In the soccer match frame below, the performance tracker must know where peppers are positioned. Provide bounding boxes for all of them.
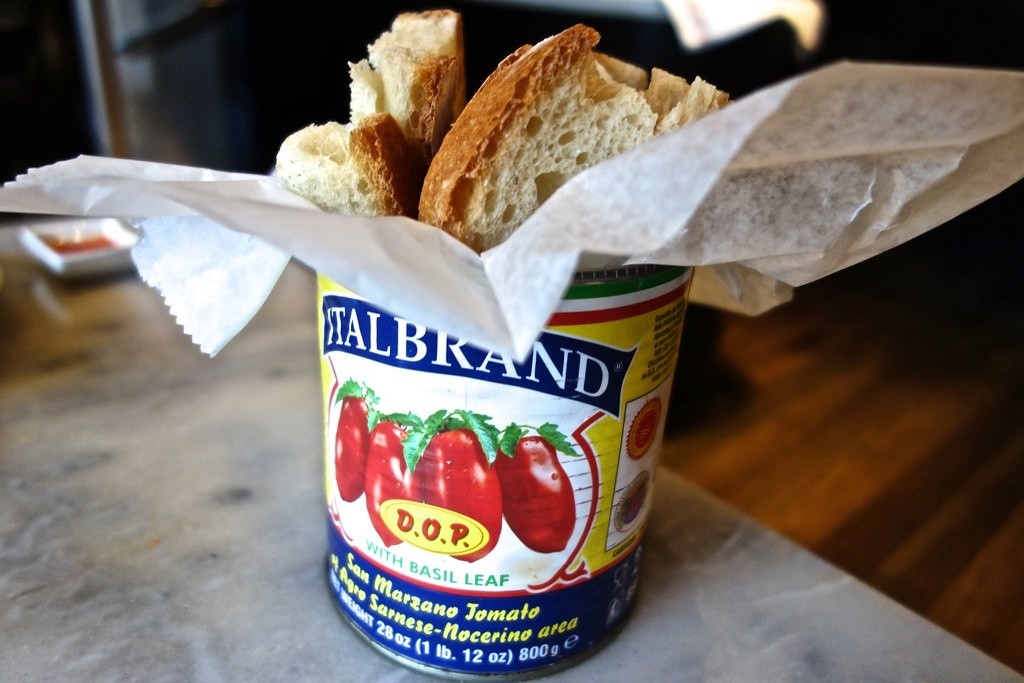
[335,396,576,562]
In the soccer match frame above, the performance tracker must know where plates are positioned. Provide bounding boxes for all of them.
[20,217,141,275]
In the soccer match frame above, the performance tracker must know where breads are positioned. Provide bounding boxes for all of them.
[273,11,733,259]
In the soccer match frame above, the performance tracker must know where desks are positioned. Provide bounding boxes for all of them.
[0,219,1023,683]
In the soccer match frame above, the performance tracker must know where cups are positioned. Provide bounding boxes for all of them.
[316,263,693,683]
